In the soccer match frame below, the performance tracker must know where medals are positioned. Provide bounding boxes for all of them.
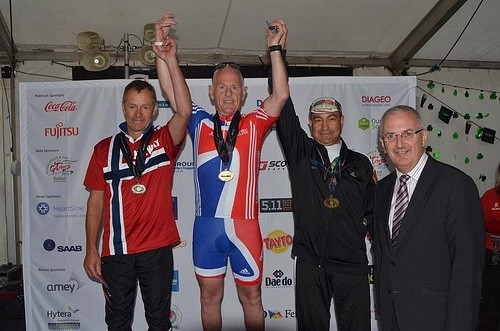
[322,196,339,208]
[218,169,233,181]
[131,183,147,194]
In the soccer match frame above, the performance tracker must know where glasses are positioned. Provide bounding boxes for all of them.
[212,61,243,81]
[381,128,424,142]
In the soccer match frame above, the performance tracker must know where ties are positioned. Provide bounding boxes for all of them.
[392,174,411,250]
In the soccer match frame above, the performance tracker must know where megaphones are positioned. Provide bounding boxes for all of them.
[77,31,111,71]
[139,23,158,65]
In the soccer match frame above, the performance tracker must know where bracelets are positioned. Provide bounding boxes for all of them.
[153,41,164,46]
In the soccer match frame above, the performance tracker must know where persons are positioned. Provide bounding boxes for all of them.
[80,36,199,331]
[479,162,500,279]
[151,11,291,331]
[364,102,485,331]
[266,16,382,331]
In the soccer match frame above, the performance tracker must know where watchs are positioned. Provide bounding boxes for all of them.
[270,44,283,53]
